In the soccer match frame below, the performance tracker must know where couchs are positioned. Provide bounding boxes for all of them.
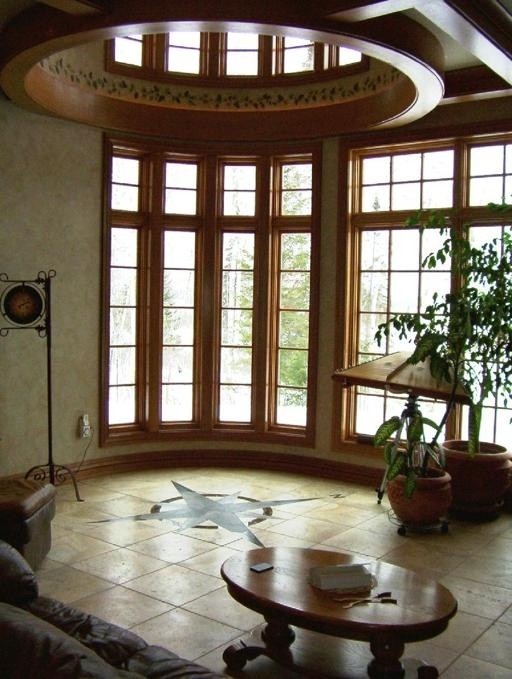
[0,538,224,677]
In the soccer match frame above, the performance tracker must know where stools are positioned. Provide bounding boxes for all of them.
[0,478,57,572]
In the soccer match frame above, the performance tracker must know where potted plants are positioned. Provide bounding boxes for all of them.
[373,408,451,538]
[372,203,512,522]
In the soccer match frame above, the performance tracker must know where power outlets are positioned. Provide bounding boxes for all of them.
[80,426,91,438]
